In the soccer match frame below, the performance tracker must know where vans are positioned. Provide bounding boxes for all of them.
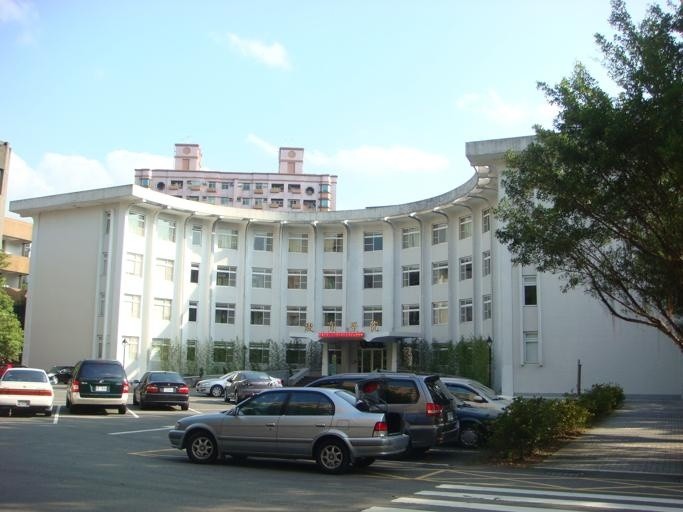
[302,372,463,461]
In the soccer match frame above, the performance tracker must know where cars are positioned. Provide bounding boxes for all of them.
[66,358,130,414]
[45,365,74,385]
[195,370,284,405]
[0,366,55,417]
[132,370,190,410]
[438,376,526,449]
[167,386,410,474]
